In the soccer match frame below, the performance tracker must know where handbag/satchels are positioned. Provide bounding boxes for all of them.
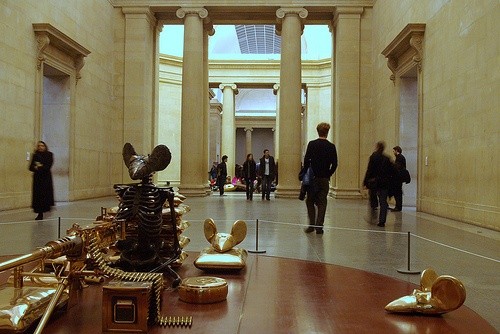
[298,173,309,201]
[404,170,412,184]
[365,174,379,188]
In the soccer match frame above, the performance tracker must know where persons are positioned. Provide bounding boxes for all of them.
[258,150,276,200]
[29,140,54,220]
[242,154,257,200]
[299,123,338,234]
[235,159,278,194]
[364,141,406,227]
[208,155,228,196]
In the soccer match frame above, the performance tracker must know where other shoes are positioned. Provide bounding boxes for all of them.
[316,227,323,234]
[377,223,386,227]
[391,207,401,211]
[305,227,314,232]
[35,212,44,220]
